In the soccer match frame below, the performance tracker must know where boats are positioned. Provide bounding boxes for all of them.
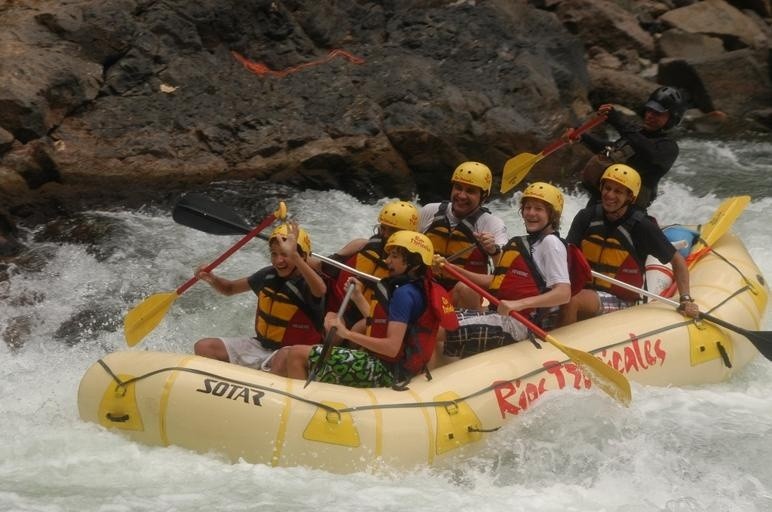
[77,224,771,479]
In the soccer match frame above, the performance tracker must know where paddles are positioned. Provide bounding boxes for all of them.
[304,277,356,389]
[124,201,288,348]
[591,269,772,367]
[432,254,632,411]
[501,109,609,195]
[173,192,383,287]
[650,195,750,303]
[445,239,481,267]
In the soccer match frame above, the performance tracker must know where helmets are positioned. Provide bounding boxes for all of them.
[600,163,642,198]
[269,225,310,261]
[377,202,423,234]
[520,182,564,215]
[451,161,492,197]
[646,86,683,129]
[384,231,433,266]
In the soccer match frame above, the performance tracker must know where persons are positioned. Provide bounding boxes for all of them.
[307,201,420,347]
[558,163,699,329]
[193,218,327,378]
[418,161,508,311]
[561,86,685,215]
[271,230,459,388]
[431,182,571,368]
